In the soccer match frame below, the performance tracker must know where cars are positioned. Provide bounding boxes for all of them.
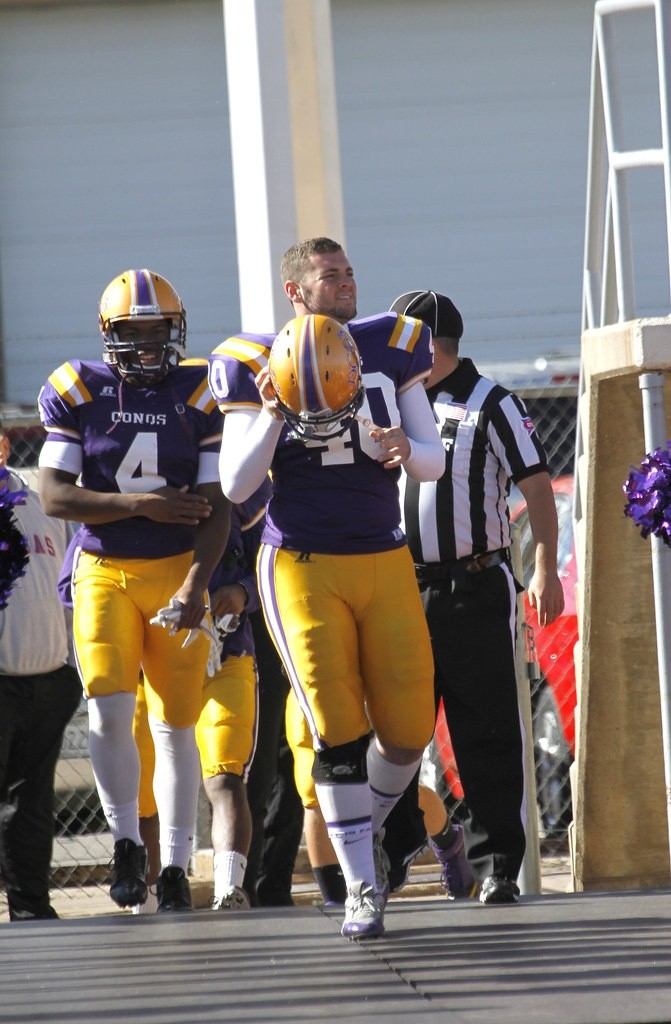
[505,475,577,621]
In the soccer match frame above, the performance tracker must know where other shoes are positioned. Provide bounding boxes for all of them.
[398,808,429,865]
[477,873,520,904]
[391,856,414,891]
[7,900,59,922]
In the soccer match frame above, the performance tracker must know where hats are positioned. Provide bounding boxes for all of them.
[389,289,464,339]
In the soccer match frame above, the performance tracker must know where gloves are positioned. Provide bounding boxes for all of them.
[150,598,224,678]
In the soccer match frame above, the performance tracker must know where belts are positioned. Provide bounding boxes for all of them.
[414,550,507,582]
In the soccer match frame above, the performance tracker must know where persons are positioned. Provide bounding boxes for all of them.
[0,268,565,922]
[206,237,446,942]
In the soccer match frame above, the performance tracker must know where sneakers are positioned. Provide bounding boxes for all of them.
[371,827,391,904]
[341,883,383,940]
[208,885,251,910]
[107,839,148,908]
[321,899,344,912]
[433,825,476,899]
[149,865,194,912]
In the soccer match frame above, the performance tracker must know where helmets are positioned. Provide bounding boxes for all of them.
[97,269,187,387]
[268,314,364,440]
